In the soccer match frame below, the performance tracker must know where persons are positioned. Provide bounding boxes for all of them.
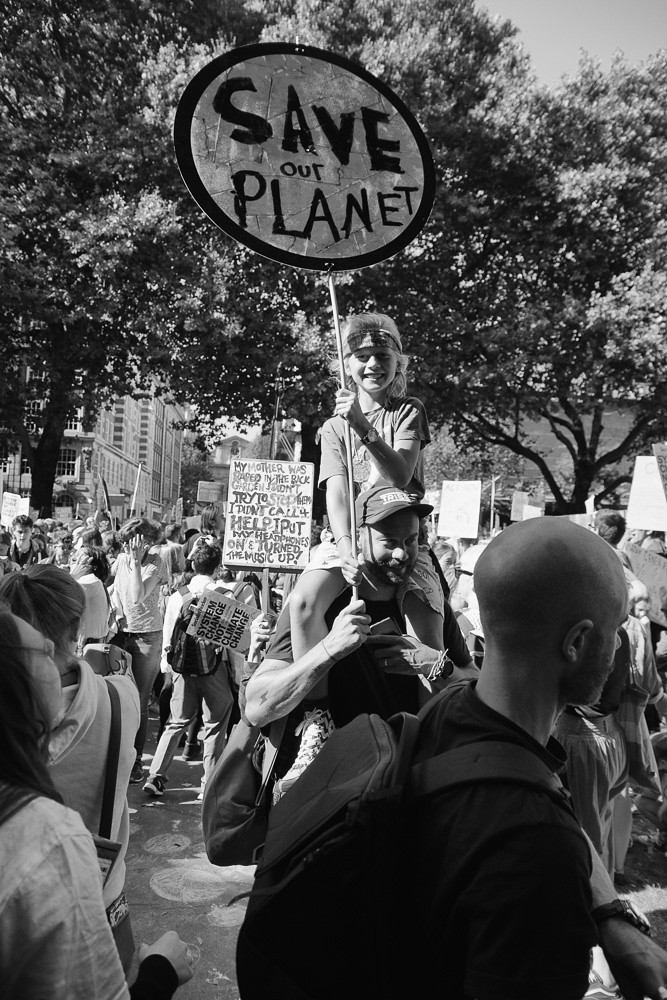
[232,518,629,1000]
[1,606,194,1000]
[2,502,652,993]
[270,310,447,810]
[1,564,146,988]
[237,481,482,814]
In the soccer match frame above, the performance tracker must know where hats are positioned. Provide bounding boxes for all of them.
[355,486,434,528]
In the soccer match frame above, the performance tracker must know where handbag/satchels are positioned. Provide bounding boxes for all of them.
[201,719,270,866]
[614,657,663,795]
[91,833,124,891]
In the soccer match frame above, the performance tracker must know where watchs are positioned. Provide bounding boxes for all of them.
[593,899,652,938]
[432,650,456,683]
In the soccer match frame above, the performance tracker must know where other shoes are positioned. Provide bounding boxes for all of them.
[182,740,200,761]
[130,761,144,782]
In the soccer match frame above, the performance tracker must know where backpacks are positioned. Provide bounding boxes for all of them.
[166,588,234,678]
[235,681,574,1000]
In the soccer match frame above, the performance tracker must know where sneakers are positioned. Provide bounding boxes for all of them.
[273,707,339,794]
[142,778,165,795]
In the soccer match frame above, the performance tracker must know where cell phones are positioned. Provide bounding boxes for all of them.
[369,617,403,647]
